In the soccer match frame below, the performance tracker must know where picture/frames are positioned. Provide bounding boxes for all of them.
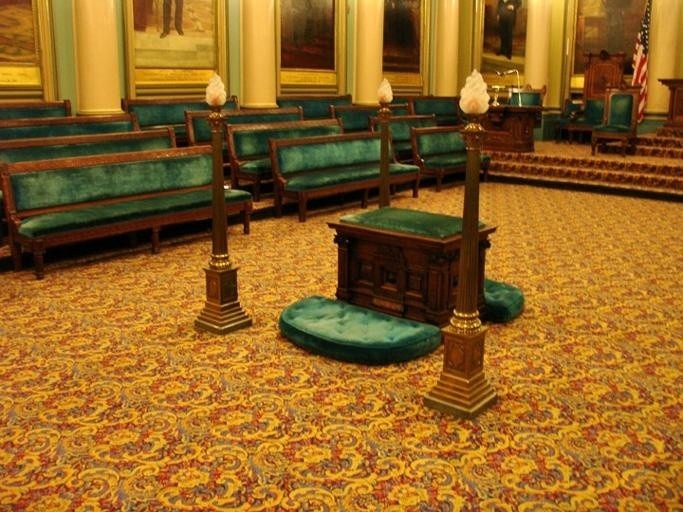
[571,0,645,78]
[1,0,61,106]
[274,0,346,98]
[381,0,430,97]
[477,0,529,85]
[123,0,230,102]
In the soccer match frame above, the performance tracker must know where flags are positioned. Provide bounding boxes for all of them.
[631,1,651,126]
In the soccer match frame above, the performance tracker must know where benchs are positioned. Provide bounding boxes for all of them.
[120,92,489,224]
[0,98,253,281]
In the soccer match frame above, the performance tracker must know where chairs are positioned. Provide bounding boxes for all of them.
[506,84,639,159]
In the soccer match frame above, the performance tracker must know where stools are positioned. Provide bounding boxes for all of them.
[327,205,497,329]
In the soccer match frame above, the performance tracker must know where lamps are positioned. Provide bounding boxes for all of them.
[192,71,253,336]
[420,69,498,418]
[497,68,522,106]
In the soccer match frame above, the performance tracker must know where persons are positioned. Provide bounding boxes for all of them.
[496,0,522,60]
[159,0,185,38]
